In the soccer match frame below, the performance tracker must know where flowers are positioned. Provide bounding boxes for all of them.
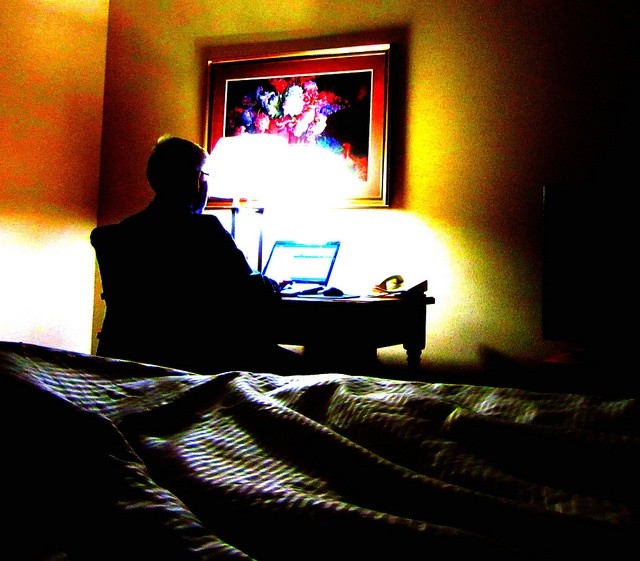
[232,81,355,146]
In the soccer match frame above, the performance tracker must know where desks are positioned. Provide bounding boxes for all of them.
[284,292,437,375]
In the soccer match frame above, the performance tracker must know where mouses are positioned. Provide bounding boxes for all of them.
[322,287,343,296]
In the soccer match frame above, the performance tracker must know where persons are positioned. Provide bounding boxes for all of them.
[117,137,302,376]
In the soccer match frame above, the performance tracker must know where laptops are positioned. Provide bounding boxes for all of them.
[264,239,341,297]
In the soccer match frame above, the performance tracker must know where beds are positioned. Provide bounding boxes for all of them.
[0,341,638,555]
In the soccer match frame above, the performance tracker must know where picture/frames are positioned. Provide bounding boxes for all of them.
[201,43,394,212]
